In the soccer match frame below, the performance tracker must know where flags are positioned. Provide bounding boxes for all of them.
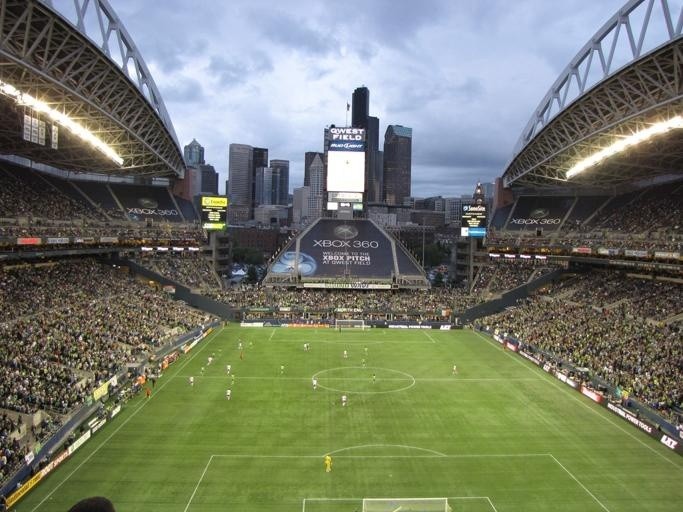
[347,104,350,111]
